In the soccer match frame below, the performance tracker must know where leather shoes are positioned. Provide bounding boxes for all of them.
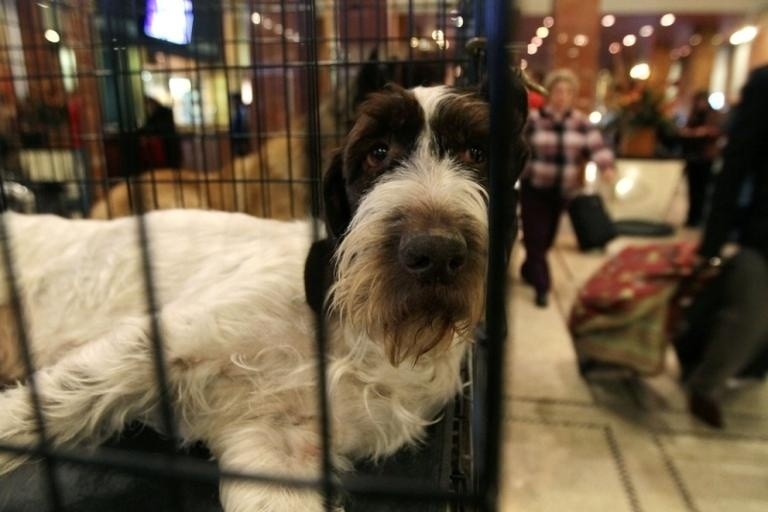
[534,289,546,307]
[688,389,728,433]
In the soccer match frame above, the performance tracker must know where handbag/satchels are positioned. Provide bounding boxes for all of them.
[563,192,618,254]
[566,243,697,379]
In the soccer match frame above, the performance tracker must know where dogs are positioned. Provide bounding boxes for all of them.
[0,74,531,512]
[90,77,354,221]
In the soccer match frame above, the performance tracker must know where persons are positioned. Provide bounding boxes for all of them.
[134,96,188,168]
[228,92,254,158]
[518,66,768,430]
